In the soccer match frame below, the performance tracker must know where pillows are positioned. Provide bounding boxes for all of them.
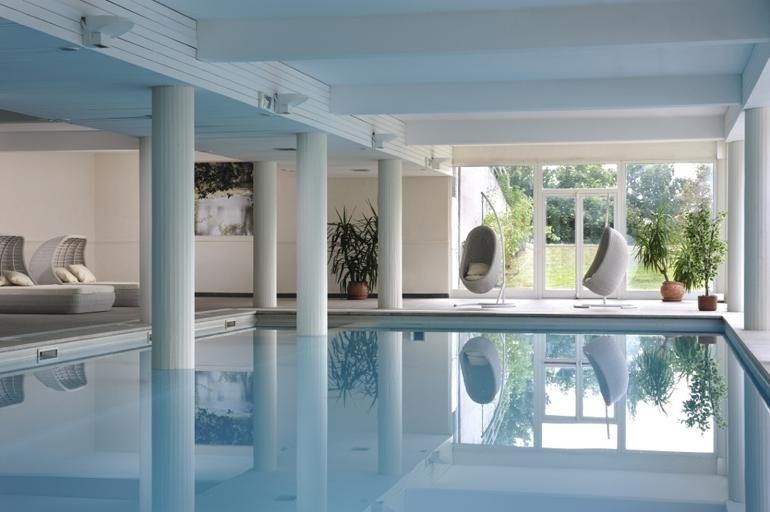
[466,260,488,280]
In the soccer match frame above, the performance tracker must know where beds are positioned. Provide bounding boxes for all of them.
[0,233,140,314]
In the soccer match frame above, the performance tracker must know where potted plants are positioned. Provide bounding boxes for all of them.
[633,203,728,311]
[678,334,730,435]
[327,196,379,300]
